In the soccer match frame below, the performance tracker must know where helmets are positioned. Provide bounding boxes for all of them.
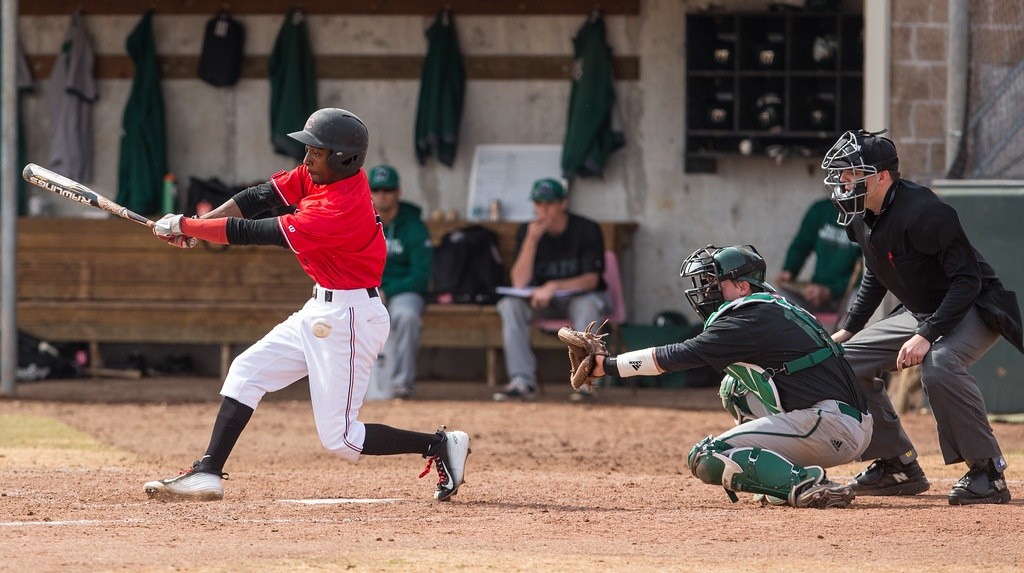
[827,130,897,170]
[706,245,776,292]
[288,108,368,179]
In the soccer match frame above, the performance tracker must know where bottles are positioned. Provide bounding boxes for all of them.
[162,173,177,215]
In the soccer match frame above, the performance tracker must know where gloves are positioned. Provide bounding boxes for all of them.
[165,215,205,249]
[153,214,183,241]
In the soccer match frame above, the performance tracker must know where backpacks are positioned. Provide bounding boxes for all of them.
[436,224,511,305]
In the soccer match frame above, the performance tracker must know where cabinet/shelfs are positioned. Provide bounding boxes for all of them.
[683,5,865,175]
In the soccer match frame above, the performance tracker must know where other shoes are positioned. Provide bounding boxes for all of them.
[494,378,539,402]
[571,388,601,404]
[391,384,415,401]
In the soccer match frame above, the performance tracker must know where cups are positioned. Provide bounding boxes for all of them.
[196,202,212,217]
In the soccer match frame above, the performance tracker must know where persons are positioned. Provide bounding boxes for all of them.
[590,244,874,510]
[822,128,1024,505]
[493,179,611,400]
[775,198,863,332]
[368,165,434,399]
[143,108,471,502]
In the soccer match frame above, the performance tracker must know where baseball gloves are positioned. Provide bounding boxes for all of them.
[557,326,611,388]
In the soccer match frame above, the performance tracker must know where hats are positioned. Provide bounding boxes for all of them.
[368,166,399,189]
[531,179,562,202]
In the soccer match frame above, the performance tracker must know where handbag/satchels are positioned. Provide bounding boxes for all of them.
[975,290,1024,354]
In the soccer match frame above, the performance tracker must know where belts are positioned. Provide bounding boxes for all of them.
[312,285,380,303]
[836,402,862,424]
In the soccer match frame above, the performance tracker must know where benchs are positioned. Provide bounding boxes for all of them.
[12,217,638,382]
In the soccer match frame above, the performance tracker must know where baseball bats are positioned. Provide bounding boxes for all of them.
[22,162,197,249]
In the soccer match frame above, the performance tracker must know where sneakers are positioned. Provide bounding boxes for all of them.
[144,460,223,501]
[849,456,929,496]
[422,431,469,499]
[798,481,855,508]
[947,463,1011,505]
